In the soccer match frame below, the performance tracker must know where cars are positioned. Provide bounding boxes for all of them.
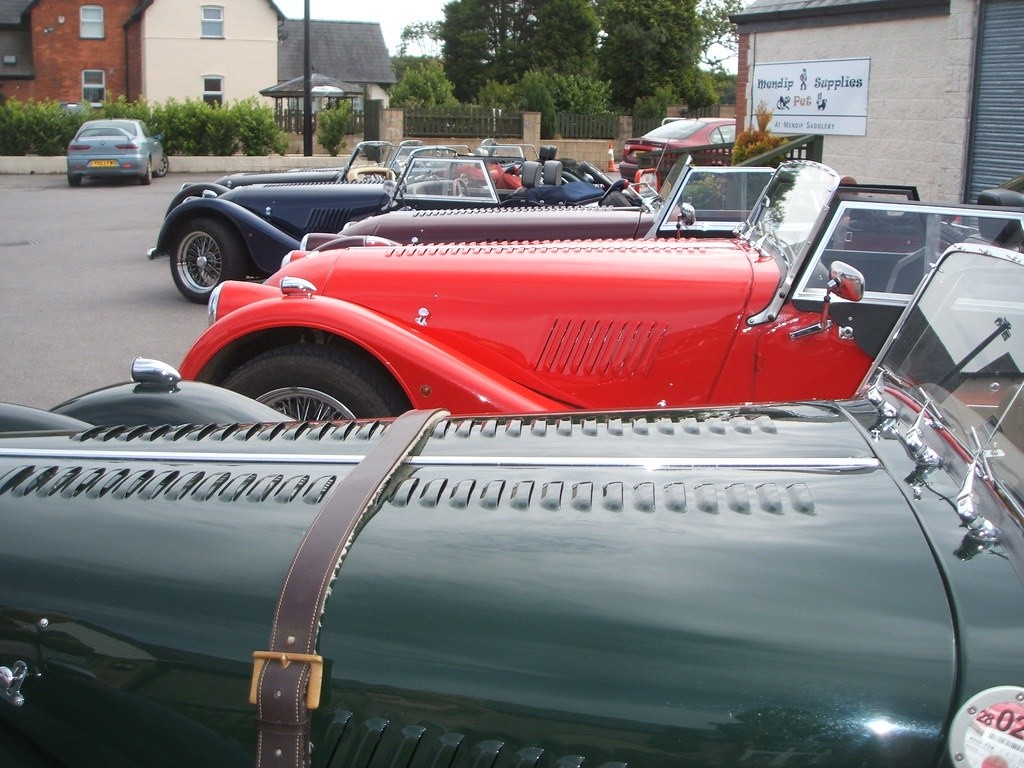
[618,118,735,182]
[1,139,1024,768]
[66,119,169,188]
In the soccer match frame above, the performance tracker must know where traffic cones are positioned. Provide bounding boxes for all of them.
[607,142,617,172]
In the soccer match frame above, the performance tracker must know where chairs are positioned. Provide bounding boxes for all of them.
[965,188,1024,253]
[515,145,563,195]
